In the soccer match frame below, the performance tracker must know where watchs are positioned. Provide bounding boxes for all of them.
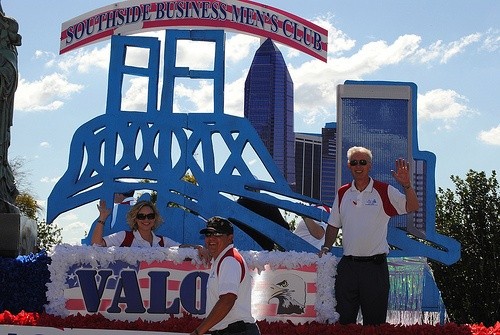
[195,329,200,335]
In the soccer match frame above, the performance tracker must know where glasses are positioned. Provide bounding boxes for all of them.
[136,213,155,220]
[205,232,224,237]
[350,159,367,166]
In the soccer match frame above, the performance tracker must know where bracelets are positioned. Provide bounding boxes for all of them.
[321,245,330,252]
[97,220,105,225]
[402,181,411,190]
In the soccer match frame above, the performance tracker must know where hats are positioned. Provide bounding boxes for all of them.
[199,216,233,234]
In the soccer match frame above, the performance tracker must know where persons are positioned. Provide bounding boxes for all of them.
[292,203,330,251]
[92,199,213,263]
[227,176,289,251]
[110,191,137,229]
[316,146,420,326]
[190,216,260,335]
